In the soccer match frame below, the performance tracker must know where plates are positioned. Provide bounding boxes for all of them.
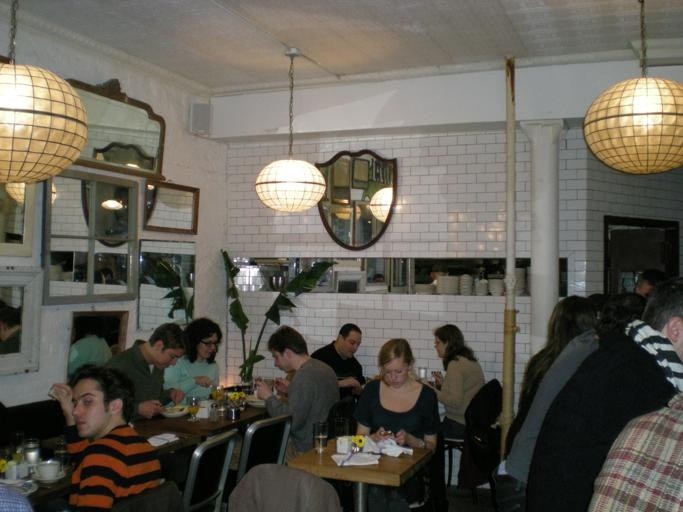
[31,472,66,484]
[437,276,459,295]
[414,284,435,294]
[0,479,39,495]
[246,398,269,408]
[160,405,188,419]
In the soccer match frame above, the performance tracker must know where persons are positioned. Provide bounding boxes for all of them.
[1,305,22,353]
[588,294,611,313]
[633,268,669,299]
[504,295,597,455]
[356,339,440,512]
[488,292,648,512]
[428,259,451,285]
[163,317,223,405]
[255,325,340,449]
[605,293,646,332]
[49,363,163,512]
[310,323,366,400]
[103,322,191,420]
[588,393,683,512]
[373,274,384,282]
[419,323,486,435]
[524,277,682,512]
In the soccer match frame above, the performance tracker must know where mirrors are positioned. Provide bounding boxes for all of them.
[142,178,199,235]
[0,183,35,257]
[62,78,166,181]
[40,168,140,307]
[137,238,197,332]
[79,180,129,248]
[313,148,398,252]
[90,142,154,172]
[144,183,158,225]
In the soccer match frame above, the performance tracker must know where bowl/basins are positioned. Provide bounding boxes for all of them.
[515,267,526,296]
[459,274,473,296]
[488,279,505,296]
[474,279,488,296]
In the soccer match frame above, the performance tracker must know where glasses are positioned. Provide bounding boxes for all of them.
[195,341,221,347]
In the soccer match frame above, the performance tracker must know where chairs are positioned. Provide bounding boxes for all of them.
[239,411,292,491]
[443,378,502,507]
[228,464,342,510]
[178,429,241,511]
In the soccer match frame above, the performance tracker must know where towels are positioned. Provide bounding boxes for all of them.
[147,433,180,447]
[331,434,413,467]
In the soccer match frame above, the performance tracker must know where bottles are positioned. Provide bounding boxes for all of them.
[210,403,218,421]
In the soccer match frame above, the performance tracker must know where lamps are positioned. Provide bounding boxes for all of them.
[584,3,683,175]
[255,46,326,214]
[0,0,87,185]
[5,175,59,208]
[368,186,396,222]
[100,197,123,209]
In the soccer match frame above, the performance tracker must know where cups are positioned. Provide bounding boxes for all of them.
[418,366,427,382]
[188,393,202,423]
[262,378,274,396]
[314,422,329,454]
[23,438,40,482]
[36,461,62,479]
